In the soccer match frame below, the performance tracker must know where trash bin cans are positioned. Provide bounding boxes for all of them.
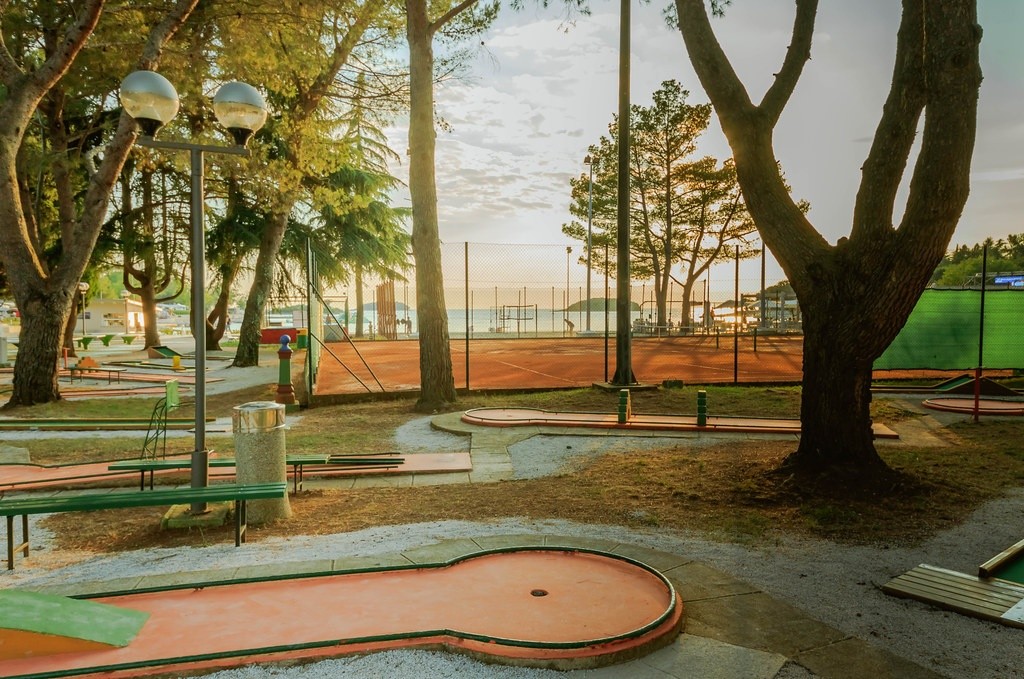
[295,328,308,350]
[233,401,293,521]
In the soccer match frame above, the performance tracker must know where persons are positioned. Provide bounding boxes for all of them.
[406,316,412,337]
[711,307,715,322]
[325,314,332,325]
[565,319,574,337]
[738,309,802,334]
[225,314,233,334]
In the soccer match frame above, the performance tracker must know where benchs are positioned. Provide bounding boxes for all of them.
[0,481,289,570]
[63,367,128,385]
[108,454,333,497]
